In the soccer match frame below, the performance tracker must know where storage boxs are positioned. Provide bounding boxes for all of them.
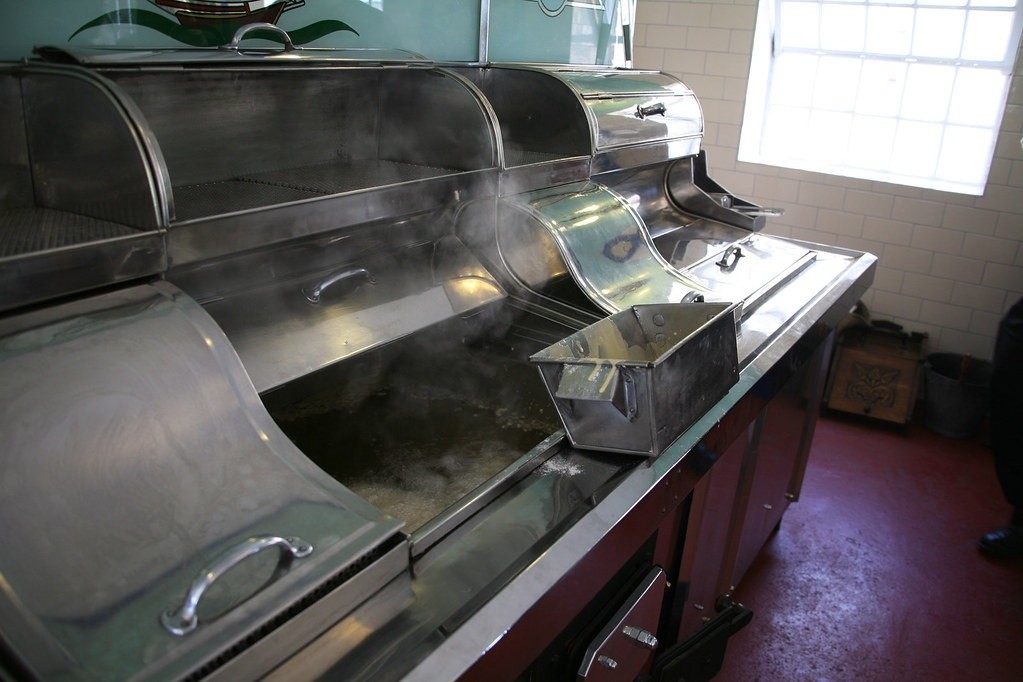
[822,318,927,436]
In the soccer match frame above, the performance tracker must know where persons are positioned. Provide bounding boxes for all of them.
[972,300,1022,565]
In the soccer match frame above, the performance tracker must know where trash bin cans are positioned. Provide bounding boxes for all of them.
[923,351,1004,438]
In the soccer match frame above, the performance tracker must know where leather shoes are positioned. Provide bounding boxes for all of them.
[979,528,1022,557]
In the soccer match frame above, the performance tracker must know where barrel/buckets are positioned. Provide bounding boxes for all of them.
[922,349,997,440]
